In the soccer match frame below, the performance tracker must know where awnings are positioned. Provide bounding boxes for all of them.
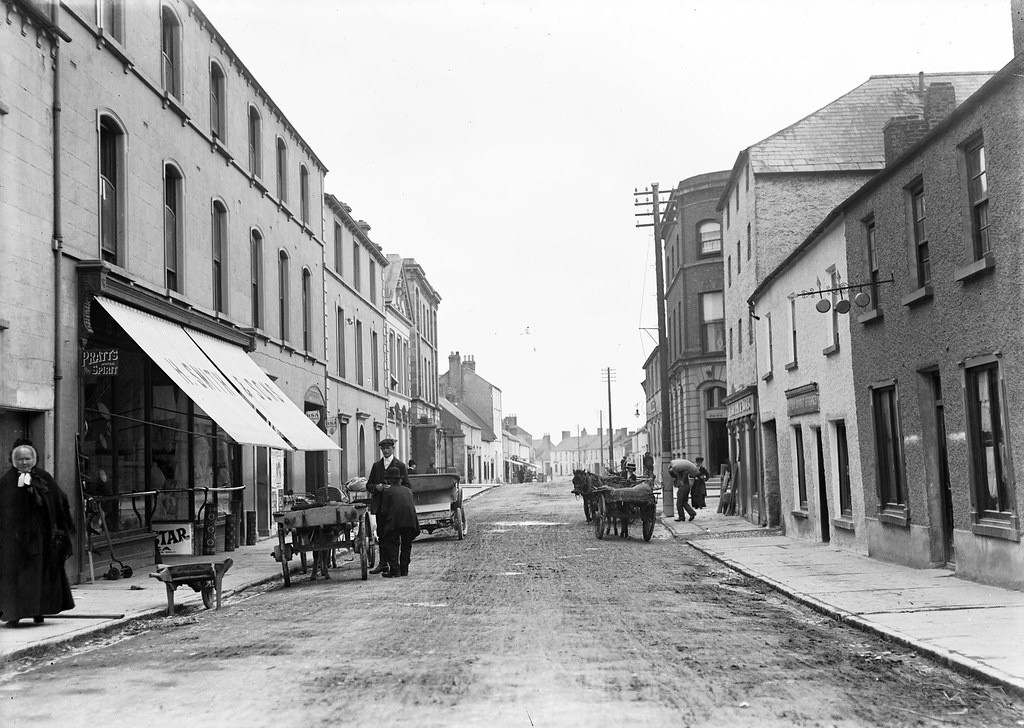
[184,328,345,452]
[518,459,538,467]
[93,296,294,450]
[505,460,524,466]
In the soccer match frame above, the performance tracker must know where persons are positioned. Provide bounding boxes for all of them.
[605,451,710,521]
[427,463,437,473]
[0,445,76,626]
[407,460,417,474]
[367,437,411,576]
[381,465,420,577]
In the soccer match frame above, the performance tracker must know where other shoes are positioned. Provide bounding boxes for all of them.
[5,620,19,628]
[32,615,45,625]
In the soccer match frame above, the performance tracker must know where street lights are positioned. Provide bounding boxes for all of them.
[600,367,616,473]
[594,409,606,466]
[575,423,582,471]
[632,183,680,517]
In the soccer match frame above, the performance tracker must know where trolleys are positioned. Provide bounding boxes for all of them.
[149,559,234,615]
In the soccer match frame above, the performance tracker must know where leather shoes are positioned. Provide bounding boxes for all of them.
[401,567,409,576]
[382,568,401,578]
[689,512,697,521]
[673,516,685,522]
[369,563,389,574]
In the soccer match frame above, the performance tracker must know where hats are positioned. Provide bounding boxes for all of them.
[645,452,650,455]
[408,460,417,465]
[378,439,398,446]
[624,463,637,469]
[695,457,704,463]
[384,467,404,478]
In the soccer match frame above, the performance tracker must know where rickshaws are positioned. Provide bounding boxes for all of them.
[402,473,469,540]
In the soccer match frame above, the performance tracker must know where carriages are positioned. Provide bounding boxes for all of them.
[270,487,376,587]
[570,468,657,524]
[591,466,657,542]
